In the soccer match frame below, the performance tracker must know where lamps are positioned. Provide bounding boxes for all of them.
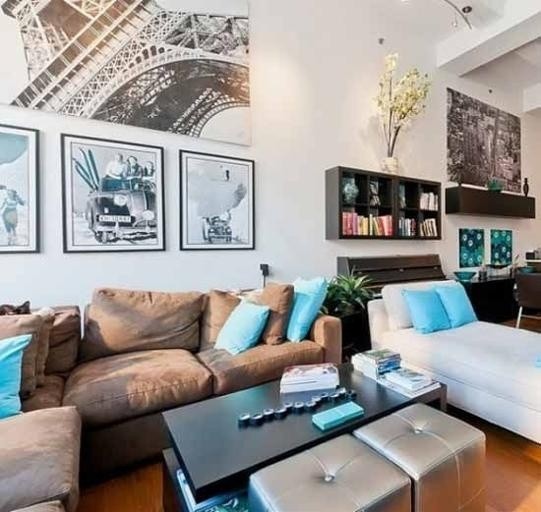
[443,0,474,30]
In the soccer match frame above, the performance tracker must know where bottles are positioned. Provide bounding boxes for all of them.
[480,263,487,280]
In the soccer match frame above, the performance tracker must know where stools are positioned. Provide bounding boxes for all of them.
[355,395,495,512]
[242,433,415,512]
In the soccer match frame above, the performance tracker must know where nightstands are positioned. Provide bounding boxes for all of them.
[330,313,358,360]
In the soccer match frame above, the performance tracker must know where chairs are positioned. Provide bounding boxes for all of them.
[510,267,541,332]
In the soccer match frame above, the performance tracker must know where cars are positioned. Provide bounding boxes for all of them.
[202,216,233,242]
[85,177,157,244]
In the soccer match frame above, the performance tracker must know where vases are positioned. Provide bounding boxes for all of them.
[379,155,403,176]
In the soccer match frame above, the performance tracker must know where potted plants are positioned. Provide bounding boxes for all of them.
[326,273,368,317]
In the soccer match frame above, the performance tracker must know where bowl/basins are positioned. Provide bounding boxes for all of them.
[453,271,476,281]
[519,265,536,274]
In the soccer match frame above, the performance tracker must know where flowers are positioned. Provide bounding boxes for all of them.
[372,52,432,154]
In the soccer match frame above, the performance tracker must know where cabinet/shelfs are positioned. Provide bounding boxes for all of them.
[462,274,536,326]
[322,165,445,243]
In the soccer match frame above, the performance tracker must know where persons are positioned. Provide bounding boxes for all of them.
[0,185,28,248]
[104,151,129,179]
[126,154,144,179]
[144,160,155,177]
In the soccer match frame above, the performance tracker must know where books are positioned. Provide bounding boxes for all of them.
[279,345,445,402]
[343,207,441,240]
[421,192,441,212]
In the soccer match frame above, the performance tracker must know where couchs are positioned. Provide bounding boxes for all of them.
[16,273,352,480]
[0,405,88,512]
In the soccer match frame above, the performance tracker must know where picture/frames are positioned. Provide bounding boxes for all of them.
[178,147,258,254]
[57,129,166,254]
[0,121,46,257]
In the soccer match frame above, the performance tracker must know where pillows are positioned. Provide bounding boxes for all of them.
[287,273,330,346]
[381,278,459,334]
[435,282,479,326]
[402,288,452,334]
[32,306,56,391]
[0,314,48,400]
[215,298,271,354]
[259,282,295,345]
[0,334,34,423]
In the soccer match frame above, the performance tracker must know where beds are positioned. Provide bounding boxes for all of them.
[335,253,541,449]
